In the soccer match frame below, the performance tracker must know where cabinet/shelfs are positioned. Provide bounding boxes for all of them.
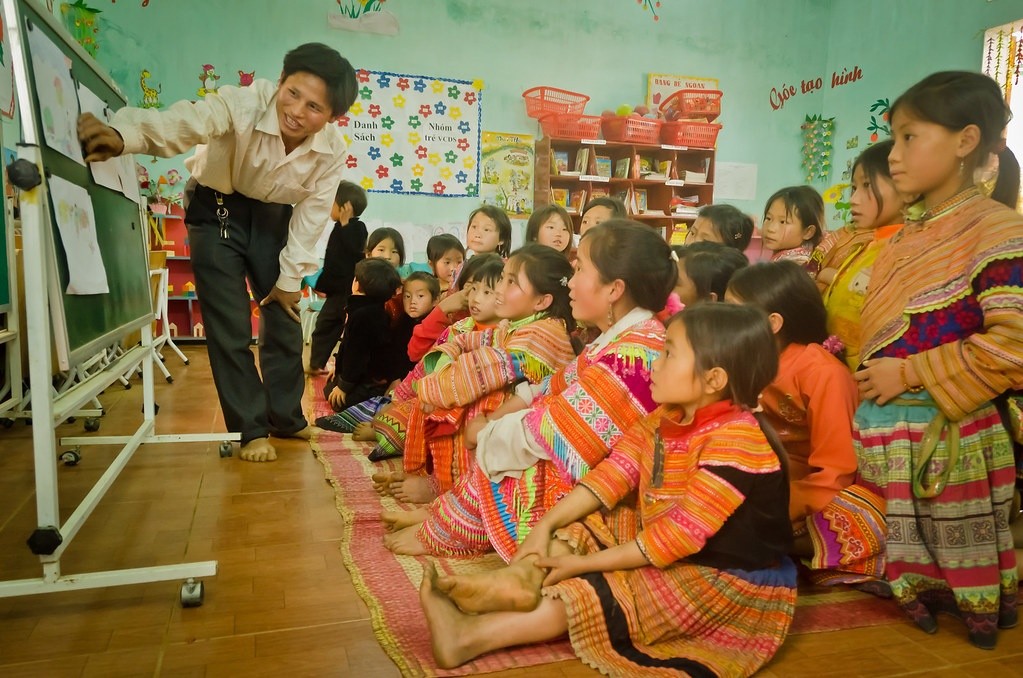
[153,214,259,340]
[533,134,715,245]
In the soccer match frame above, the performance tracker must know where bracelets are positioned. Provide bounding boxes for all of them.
[900,354,924,394]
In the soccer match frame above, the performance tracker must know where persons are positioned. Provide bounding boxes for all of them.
[303,139,925,678]
[75,42,358,461]
[379,217,682,558]
[849,71,1021,651]
[419,301,800,678]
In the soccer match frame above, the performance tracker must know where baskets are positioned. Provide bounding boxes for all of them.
[522,86,723,148]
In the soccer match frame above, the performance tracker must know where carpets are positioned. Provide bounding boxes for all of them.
[304,361,1023,678]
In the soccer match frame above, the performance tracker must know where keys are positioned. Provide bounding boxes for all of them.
[216,218,230,239]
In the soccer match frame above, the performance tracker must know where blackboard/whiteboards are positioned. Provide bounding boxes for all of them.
[0,111,12,314]
[4,1,156,371]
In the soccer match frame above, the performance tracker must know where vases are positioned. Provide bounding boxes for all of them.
[148,205,167,215]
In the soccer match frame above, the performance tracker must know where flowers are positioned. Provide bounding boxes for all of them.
[136,163,184,205]
